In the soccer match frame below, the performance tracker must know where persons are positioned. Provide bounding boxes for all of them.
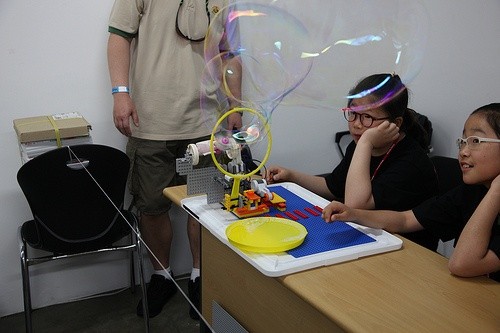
[321,101,500,284]
[262,73,440,253]
[107,0,244,320]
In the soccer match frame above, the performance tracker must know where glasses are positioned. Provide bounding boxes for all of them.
[342,107,392,128]
[455,136,500,149]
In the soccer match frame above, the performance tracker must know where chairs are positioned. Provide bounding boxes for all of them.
[405,108,441,251]
[17,144,150,333]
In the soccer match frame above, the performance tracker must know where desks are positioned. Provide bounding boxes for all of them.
[163,173,500,333]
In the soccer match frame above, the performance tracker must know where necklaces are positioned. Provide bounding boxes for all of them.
[371,136,399,182]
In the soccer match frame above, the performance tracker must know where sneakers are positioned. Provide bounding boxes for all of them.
[136,271,177,319]
[188,276,203,320]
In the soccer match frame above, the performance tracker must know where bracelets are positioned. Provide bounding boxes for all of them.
[111,86,130,94]
[229,108,243,116]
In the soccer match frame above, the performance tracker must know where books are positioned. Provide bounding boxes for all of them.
[17,133,93,166]
